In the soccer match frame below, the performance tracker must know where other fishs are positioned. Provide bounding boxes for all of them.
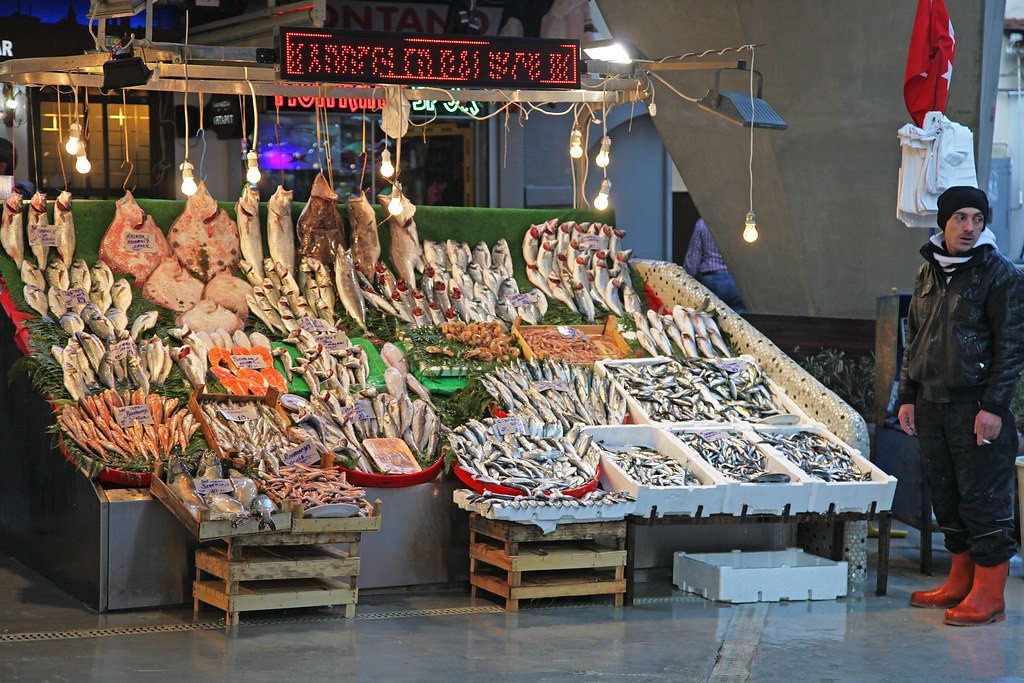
[0,172,874,533]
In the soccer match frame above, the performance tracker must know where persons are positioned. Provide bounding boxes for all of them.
[684,218,747,314]
[898,186,1024,627]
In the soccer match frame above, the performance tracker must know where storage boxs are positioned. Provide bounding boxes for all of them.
[673,547,849,604]
[581,353,900,519]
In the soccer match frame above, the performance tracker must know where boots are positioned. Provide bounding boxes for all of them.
[943,559,1010,627]
[910,551,975,608]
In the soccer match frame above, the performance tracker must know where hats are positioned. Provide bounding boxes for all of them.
[936,185,989,232]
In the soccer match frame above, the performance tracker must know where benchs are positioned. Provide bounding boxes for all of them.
[871,292,1024,577]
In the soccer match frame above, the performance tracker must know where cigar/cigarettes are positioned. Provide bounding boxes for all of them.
[982,438,991,443]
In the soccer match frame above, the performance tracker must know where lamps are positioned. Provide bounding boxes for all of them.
[695,66,788,130]
[101,34,154,89]
[583,39,633,64]
